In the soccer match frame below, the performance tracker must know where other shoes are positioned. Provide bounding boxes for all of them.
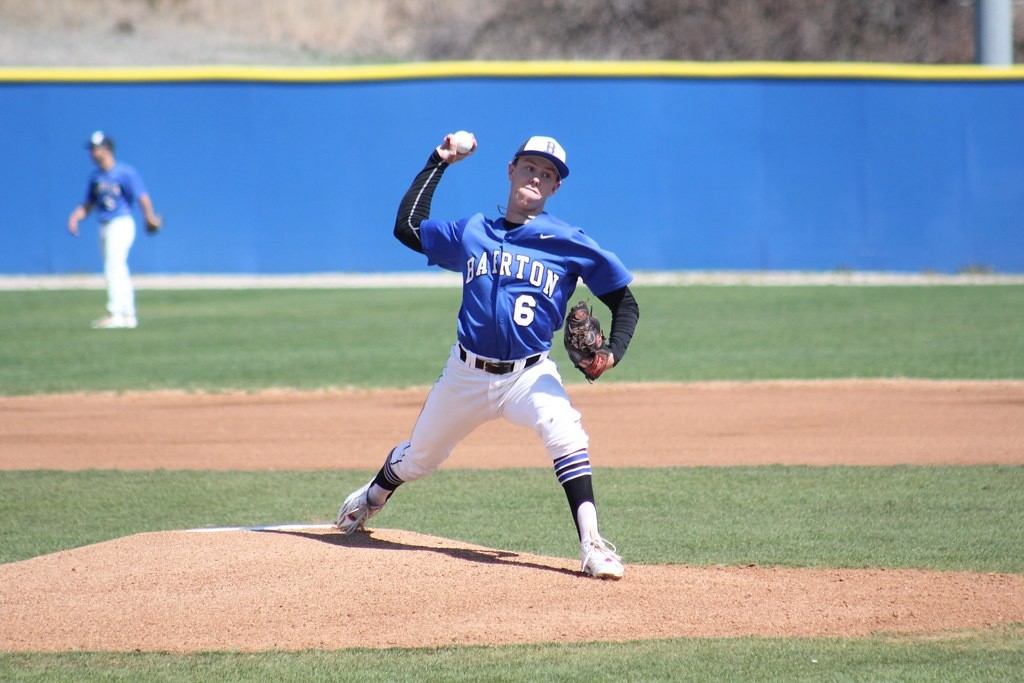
[92,313,136,329]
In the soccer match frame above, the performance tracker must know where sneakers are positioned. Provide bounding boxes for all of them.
[582,532,624,578]
[338,476,393,533]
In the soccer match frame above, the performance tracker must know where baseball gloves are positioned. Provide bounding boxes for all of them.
[562,301,611,382]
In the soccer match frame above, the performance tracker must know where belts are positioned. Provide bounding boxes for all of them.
[459,344,541,375]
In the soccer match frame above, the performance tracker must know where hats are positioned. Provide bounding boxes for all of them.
[85,132,112,148]
[515,136,569,181]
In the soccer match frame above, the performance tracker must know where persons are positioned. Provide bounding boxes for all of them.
[67,132,161,330]
[336,133,640,579]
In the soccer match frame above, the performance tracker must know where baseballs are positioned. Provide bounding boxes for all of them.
[454,130,473,154]
